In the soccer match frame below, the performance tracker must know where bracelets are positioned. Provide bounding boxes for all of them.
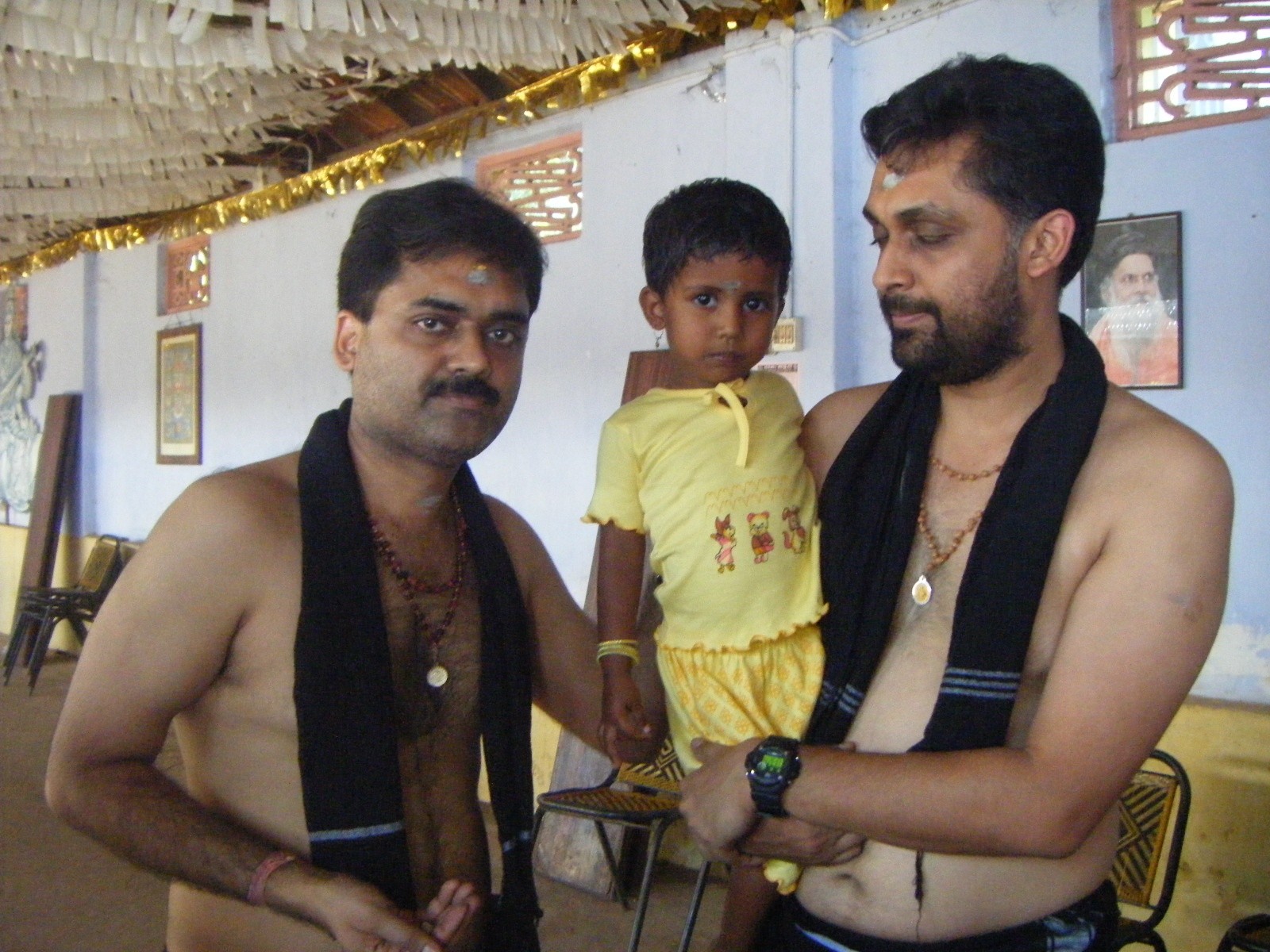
[245,849,301,909]
[596,639,642,668]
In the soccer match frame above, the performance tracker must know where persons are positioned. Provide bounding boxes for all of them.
[583,177,830,952]
[676,56,1236,952]
[1083,230,1179,387]
[44,176,663,952]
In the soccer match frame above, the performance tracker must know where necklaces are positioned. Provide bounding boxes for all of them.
[364,481,469,687]
[911,450,1003,606]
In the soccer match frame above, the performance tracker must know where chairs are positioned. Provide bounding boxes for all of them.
[529,726,711,952]
[1102,745,1193,952]
[1,531,123,695]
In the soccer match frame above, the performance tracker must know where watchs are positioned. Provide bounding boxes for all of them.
[745,735,803,819]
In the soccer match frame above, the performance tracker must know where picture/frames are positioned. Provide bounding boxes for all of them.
[155,323,204,465]
[1080,211,1185,391]
